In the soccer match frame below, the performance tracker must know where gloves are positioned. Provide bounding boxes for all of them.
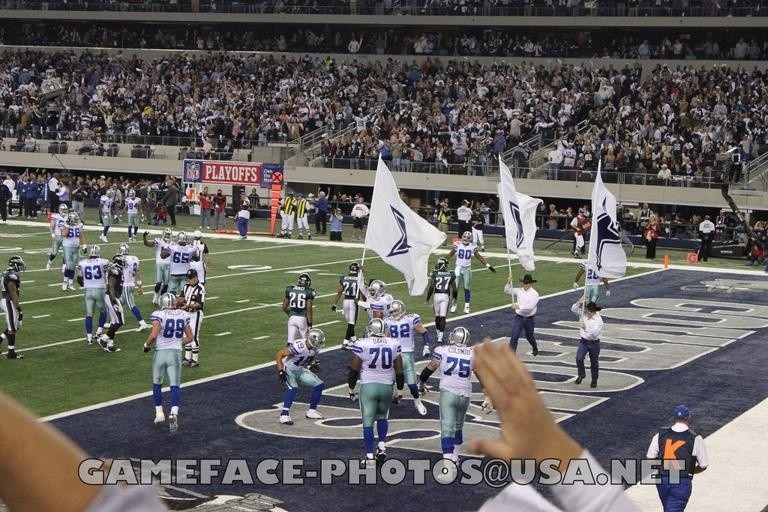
[138,282,144,294]
[144,343,150,353]
[17,308,23,321]
[394,389,402,403]
[279,370,287,384]
[331,304,336,310]
[423,345,429,356]
[482,397,493,415]
[82,244,87,253]
[113,302,120,313]
[308,323,312,331]
[349,388,358,402]
[606,289,610,298]
[486,264,495,272]
[354,297,359,303]
[425,298,429,303]
[143,231,148,237]
[574,281,578,288]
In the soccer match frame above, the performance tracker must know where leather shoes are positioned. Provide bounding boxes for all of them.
[591,381,597,387]
[575,376,584,385]
[533,346,538,356]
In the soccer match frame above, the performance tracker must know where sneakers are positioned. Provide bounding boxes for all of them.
[304,409,325,419]
[168,414,178,436]
[108,341,121,352]
[97,335,111,352]
[414,398,427,416]
[180,358,190,364]
[68,283,76,290]
[437,330,443,342]
[375,447,386,463]
[152,295,158,304]
[88,338,93,344]
[46,263,51,270]
[100,232,108,242]
[280,415,294,425]
[189,360,200,368]
[451,304,456,313]
[7,353,24,359]
[418,381,429,396]
[62,281,68,290]
[154,412,166,424]
[95,333,99,337]
[342,339,354,350]
[361,455,375,466]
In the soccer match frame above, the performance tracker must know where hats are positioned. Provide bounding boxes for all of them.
[308,193,314,198]
[519,274,537,283]
[674,404,690,421]
[661,164,667,168]
[462,200,470,204]
[585,302,601,311]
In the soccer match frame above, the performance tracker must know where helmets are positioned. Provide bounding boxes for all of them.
[462,231,472,245]
[336,208,340,211]
[186,269,196,277]
[349,262,359,275]
[59,203,68,213]
[450,327,469,345]
[129,189,136,198]
[307,329,325,349]
[368,318,385,336]
[8,255,26,272]
[159,293,177,309]
[368,280,387,297]
[178,232,187,243]
[106,189,113,196]
[299,274,310,286]
[89,245,100,258]
[68,212,79,226]
[389,301,406,319]
[162,228,172,241]
[119,244,129,254]
[194,230,202,237]
[438,257,448,271]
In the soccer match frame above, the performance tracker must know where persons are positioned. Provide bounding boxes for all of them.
[15,176,30,217]
[47,204,69,271]
[122,189,144,240]
[624,208,634,220]
[571,295,603,388]
[419,326,494,480]
[275,329,325,424]
[0,0,768,19]
[647,405,708,512]
[617,205,635,253]
[0,178,12,223]
[583,206,590,217]
[277,194,297,238]
[78,245,110,345]
[118,244,148,332]
[467,336,644,512]
[99,175,167,191]
[355,280,393,318]
[547,0,768,190]
[659,213,701,239]
[96,253,128,352]
[61,212,88,290]
[473,208,486,250]
[698,215,715,261]
[148,197,167,226]
[504,274,539,357]
[425,258,457,342]
[310,190,327,234]
[39,173,48,204]
[643,216,659,259]
[160,232,200,299]
[567,208,574,229]
[25,174,38,219]
[159,175,181,203]
[349,318,404,468]
[198,187,212,229]
[156,180,178,226]
[436,202,451,234]
[0,255,26,360]
[234,205,250,239]
[322,1,547,170]
[248,188,261,210]
[480,203,492,224]
[570,208,587,258]
[426,197,440,208]
[0,389,171,512]
[3,174,16,216]
[213,189,227,230]
[331,194,359,202]
[72,185,88,222]
[176,270,206,367]
[56,178,70,212]
[48,173,61,212]
[106,185,122,209]
[350,197,370,240]
[632,206,641,220]
[0,0,322,160]
[444,232,496,313]
[185,229,209,290]
[457,200,474,239]
[282,273,322,367]
[144,228,176,304]
[574,259,611,307]
[330,208,343,241]
[88,176,98,198]
[715,210,768,265]
[240,194,251,210]
[637,204,654,235]
[295,193,312,240]
[546,204,559,230]
[383,301,430,415]
[331,263,369,351]
[144,293,194,433]
[70,175,88,185]
[99,190,113,242]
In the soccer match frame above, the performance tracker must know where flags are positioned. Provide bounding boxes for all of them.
[498,155,544,271]
[588,160,628,279]
[366,152,448,296]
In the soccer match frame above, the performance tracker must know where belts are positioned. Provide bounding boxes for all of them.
[516,314,535,319]
[582,338,599,344]
